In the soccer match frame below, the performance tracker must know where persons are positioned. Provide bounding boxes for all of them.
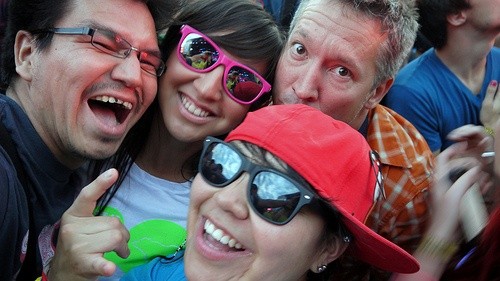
[250,180,300,221]
[202,142,230,184]
[226,65,263,102]
[96,103,421,281]
[379,0,500,159]
[269,0,436,280]
[92,0,285,281]
[0,0,168,281]
[180,46,219,69]
[393,109,500,281]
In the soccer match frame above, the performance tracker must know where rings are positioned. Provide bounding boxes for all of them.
[486,127,495,136]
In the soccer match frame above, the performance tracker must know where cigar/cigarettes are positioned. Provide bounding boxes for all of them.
[481,151,495,157]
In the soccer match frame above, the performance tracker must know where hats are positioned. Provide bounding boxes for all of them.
[223,104,421,274]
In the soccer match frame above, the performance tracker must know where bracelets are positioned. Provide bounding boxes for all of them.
[415,233,460,261]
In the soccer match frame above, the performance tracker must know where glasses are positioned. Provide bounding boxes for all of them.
[38,26,167,77]
[174,24,272,106]
[199,136,338,227]
